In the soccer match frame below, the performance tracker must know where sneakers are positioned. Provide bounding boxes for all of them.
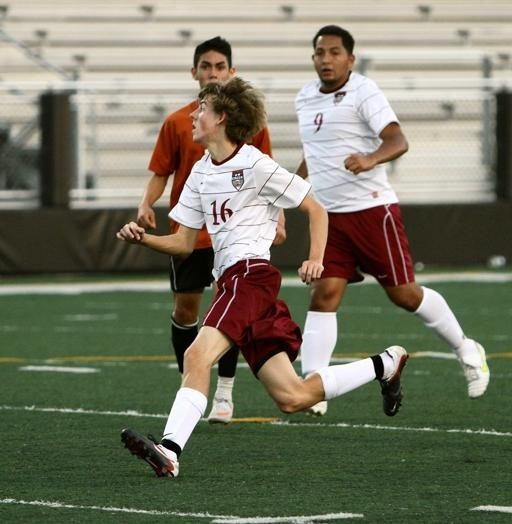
[379,341,411,419]
[456,335,493,400]
[206,393,236,426]
[118,422,183,479]
[300,399,329,418]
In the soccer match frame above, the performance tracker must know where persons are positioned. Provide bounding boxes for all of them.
[115,73,411,479]
[291,21,492,419]
[134,33,290,426]
